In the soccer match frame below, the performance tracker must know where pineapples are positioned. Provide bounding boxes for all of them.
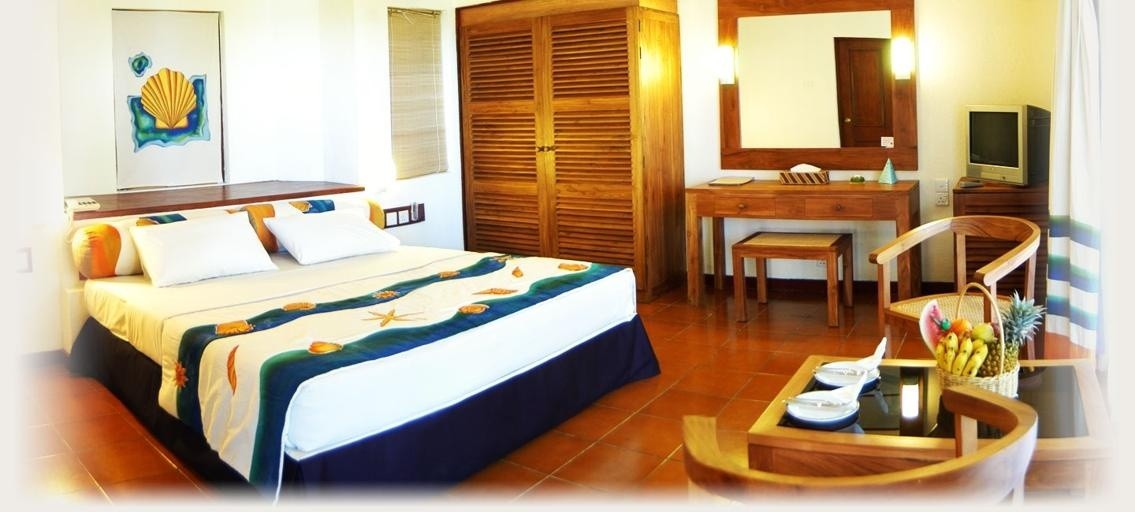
[980,291,1045,376]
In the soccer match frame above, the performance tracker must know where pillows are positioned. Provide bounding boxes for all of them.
[71,209,241,279]
[263,208,399,266]
[128,210,280,288]
[239,198,385,253]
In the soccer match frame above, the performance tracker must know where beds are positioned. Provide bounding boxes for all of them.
[66,180,659,504]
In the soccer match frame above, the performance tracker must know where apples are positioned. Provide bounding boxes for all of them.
[950,318,994,341]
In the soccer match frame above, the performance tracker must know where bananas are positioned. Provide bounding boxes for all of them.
[933,332,988,377]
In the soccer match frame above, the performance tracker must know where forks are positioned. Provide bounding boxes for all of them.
[811,364,875,377]
[781,395,852,408]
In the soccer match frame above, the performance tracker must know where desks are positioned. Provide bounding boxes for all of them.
[748,355,1112,498]
[685,179,922,307]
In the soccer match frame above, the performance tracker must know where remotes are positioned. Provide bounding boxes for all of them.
[958,178,983,186]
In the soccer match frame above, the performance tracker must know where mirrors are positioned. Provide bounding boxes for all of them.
[718,0,918,171]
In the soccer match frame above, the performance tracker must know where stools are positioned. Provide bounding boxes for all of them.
[732,232,853,328]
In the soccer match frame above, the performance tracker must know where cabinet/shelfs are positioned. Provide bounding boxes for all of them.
[456,0,685,304]
[953,177,1048,305]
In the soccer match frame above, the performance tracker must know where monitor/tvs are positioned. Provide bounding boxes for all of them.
[961,104,1051,186]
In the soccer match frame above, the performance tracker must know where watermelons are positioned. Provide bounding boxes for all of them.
[920,299,947,356]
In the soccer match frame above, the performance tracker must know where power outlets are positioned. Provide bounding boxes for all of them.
[935,193,949,206]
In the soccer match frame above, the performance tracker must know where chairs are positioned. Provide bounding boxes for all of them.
[680,385,1038,506]
[869,215,1041,360]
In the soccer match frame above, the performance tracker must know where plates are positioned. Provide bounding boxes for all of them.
[787,389,861,425]
[708,178,754,186]
[816,361,881,389]
